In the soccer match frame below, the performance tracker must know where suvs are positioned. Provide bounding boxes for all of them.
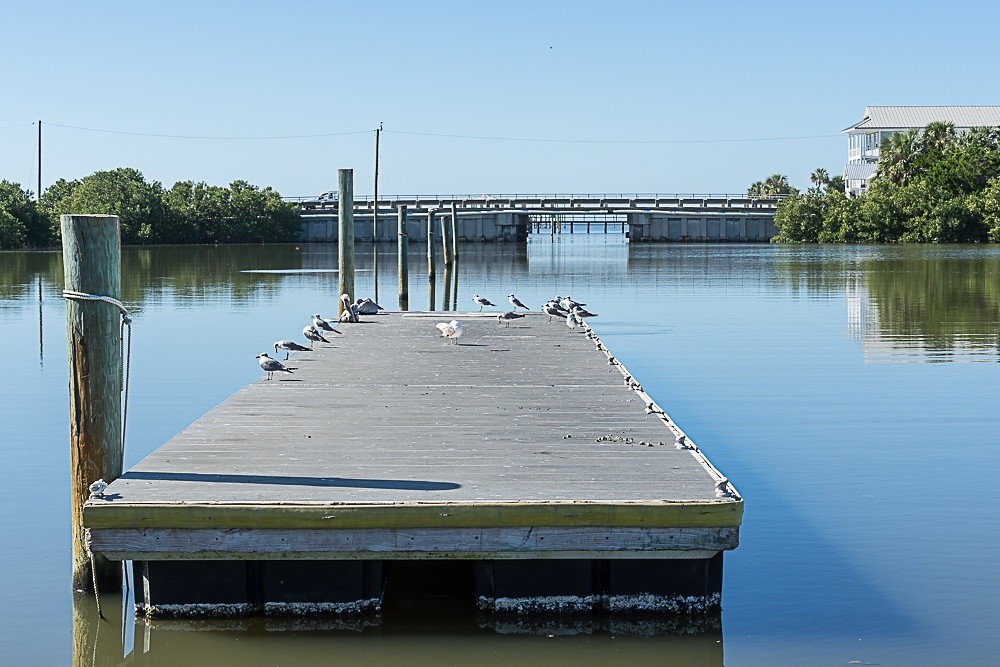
[301,191,339,210]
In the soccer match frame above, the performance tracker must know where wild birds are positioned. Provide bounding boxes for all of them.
[256,353,298,381]
[310,313,343,336]
[541,295,599,334]
[274,339,314,360]
[338,294,385,323]
[472,293,530,328]
[435,319,464,346]
[303,326,331,348]
